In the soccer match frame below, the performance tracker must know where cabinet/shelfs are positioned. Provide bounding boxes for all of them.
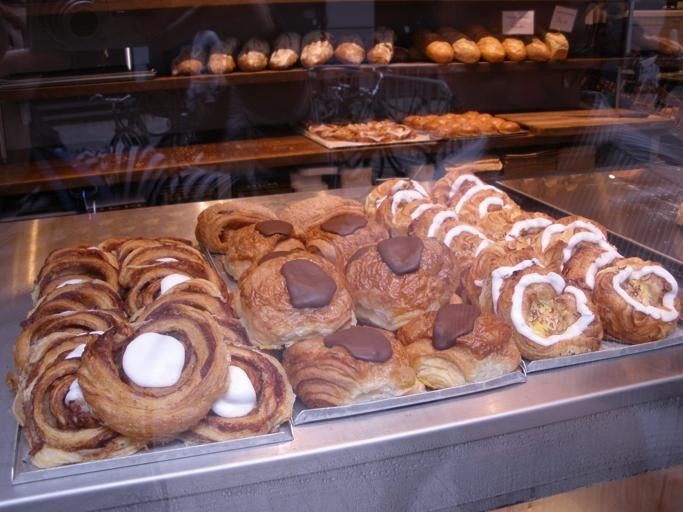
[1,0,682,502]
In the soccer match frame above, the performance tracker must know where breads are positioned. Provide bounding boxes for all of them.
[195,195,522,408]
[536,26,570,60]
[438,24,483,65]
[301,30,334,67]
[404,111,521,140]
[366,169,679,361]
[333,33,367,64]
[15,236,296,469]
[310,117,413,142]
[525,37,549,62]
[207,38,236,74]
[464,24,506,63]
[175,45,208,72]
[502,35,524,60]
[409,29,454,65]
[237,37,269,71]
[366,24,396,65]
[270,25,301,66]
